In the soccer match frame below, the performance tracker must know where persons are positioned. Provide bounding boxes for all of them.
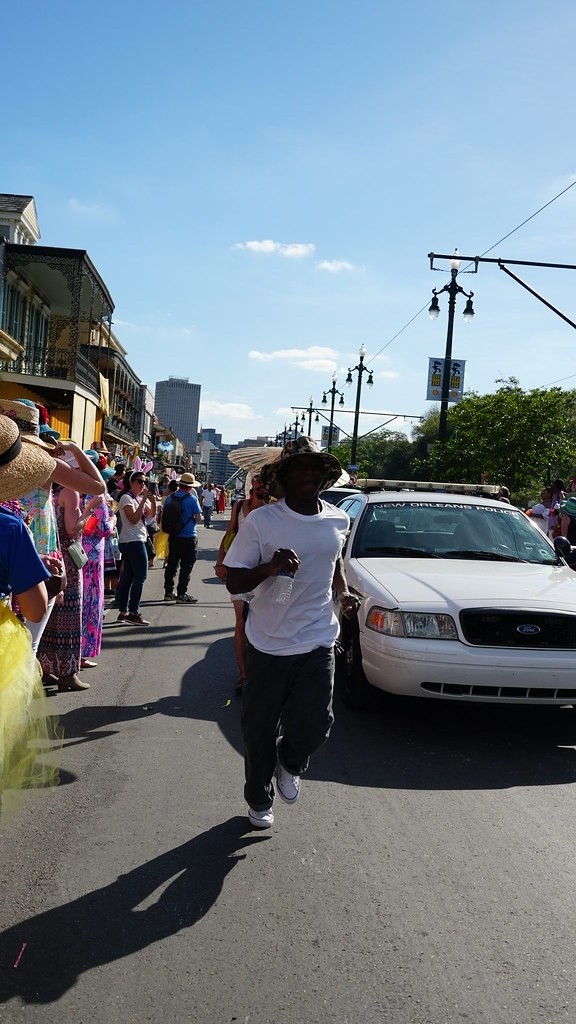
[0,413,65,820]
[0,397,230,767]
[222,436,362,827]
[214,476,277,688]
[480,470,576,545]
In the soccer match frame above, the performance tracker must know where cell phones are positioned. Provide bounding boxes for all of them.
[148,481,156,495]
[44,435,59,448]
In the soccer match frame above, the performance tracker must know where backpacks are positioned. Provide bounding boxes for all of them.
[161,492,196,534]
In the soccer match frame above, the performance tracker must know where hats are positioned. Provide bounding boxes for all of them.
[178,473,201,488]
[0,399,60,502]
[84,441,116,480]
[259,434,344,500]
[561,497,576,516]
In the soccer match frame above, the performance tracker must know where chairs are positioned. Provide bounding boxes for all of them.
[360,519,398,549]
[451,520,494,553]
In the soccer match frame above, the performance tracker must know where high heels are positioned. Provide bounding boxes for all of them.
[57,675,91,692]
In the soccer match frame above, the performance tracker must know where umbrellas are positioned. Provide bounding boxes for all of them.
[227,447,351,488]
[157,441,174,452]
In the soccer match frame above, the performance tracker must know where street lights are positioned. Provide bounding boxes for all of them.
[264,394,320,447]
[428,247,475,443]
[321,371,345,454]
[346,343,374,465]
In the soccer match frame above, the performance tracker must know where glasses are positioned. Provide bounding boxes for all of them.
[254,478,262,483]
[253,491,266,500]
[133,479,147,485]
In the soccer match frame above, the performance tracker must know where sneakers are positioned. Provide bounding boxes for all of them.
[117,612,149,626]
[275,736,300,805]
[164,591,198,604]
[248,806,275,828]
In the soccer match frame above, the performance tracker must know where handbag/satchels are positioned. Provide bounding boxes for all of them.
[146,538,156,561]
[68,540,89,569]
[223,531,237,553]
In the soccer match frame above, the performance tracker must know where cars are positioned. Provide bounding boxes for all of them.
[318,478,576,704]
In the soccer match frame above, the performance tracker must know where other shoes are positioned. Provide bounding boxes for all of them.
[81,659,98,667]
[235,677,246,693]
[103,588,116,597]
[44,673,59,685]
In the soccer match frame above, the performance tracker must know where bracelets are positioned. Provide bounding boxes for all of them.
[89,507,94,513]
[143,499,146,502]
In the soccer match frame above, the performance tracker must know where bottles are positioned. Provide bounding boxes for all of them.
[271,571,295,606]
